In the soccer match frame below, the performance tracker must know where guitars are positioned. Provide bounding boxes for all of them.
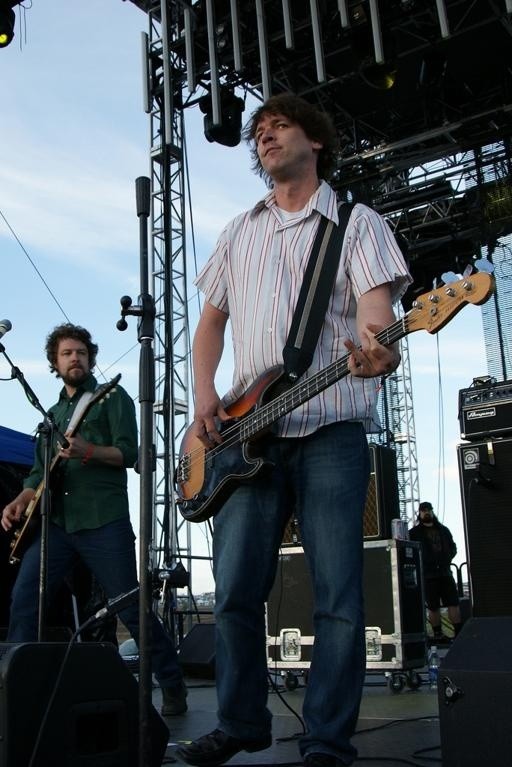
[176,274,496,521]
[8,373,123,563]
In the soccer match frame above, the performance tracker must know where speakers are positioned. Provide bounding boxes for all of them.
[459,438,511,618]
[177,624,217,682]
[437,618,512,767]
[1,642,171,765]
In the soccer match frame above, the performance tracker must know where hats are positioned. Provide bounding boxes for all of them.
[418,502,433,512]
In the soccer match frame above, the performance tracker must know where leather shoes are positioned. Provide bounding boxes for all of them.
[297,734,362,761]
[162,686,188,723]
[176,721,275,766]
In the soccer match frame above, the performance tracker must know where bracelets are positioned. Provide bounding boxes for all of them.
[81,442,95,462]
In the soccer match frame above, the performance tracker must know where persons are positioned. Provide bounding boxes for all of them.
[408,500,463,646]
[178,96,414,766]
[1,324,191,717]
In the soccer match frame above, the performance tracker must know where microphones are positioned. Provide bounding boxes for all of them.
[0,320,11,342]
[136,177,151,219]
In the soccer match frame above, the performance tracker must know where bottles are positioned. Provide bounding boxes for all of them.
[428,647,442,689]
